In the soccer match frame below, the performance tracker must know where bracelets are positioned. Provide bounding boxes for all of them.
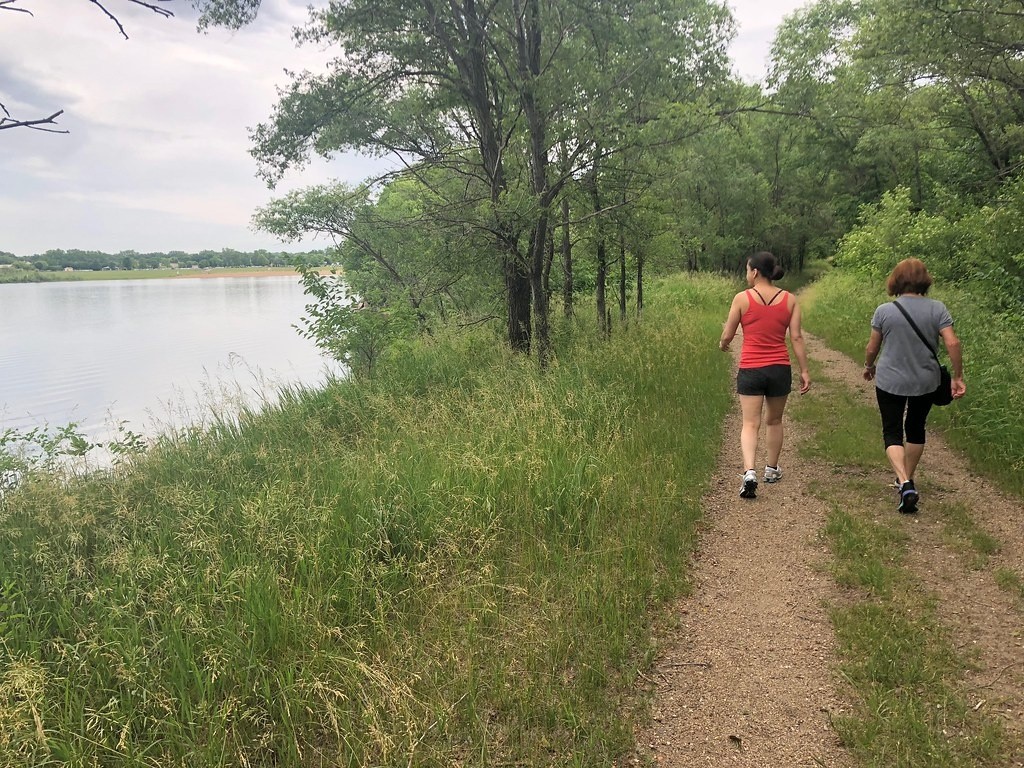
[863,361,874,369]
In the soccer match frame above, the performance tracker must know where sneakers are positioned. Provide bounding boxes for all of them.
[898,482,919,513]
[763,465,782,483]
[894,477,915,489]
[739,470,758,498]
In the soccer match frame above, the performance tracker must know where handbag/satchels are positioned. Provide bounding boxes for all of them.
[928,364,954,406]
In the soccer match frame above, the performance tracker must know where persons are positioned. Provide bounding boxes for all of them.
[719,252,811,498]
[861,257,966,512]
